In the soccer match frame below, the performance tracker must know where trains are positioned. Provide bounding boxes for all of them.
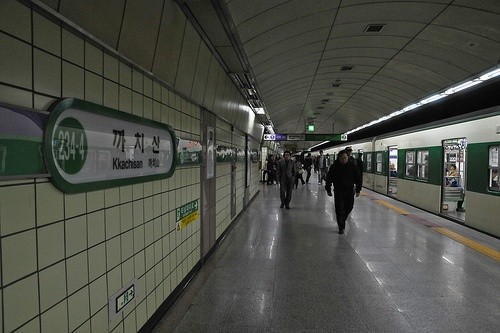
[304,105,500,239]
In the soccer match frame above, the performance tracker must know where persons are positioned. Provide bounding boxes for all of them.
[325,151,363,235]
[261,147,500,212]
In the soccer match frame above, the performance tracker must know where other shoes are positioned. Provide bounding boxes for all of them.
[318,182,321,184]
[456,207,465,212]
[285,203,290,209]
[339,228,344,234]
[280,202,284,208]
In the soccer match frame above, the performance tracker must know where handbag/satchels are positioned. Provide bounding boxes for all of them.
[298,169,304,174]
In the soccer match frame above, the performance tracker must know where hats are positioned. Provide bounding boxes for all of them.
[345,147,353,152]
